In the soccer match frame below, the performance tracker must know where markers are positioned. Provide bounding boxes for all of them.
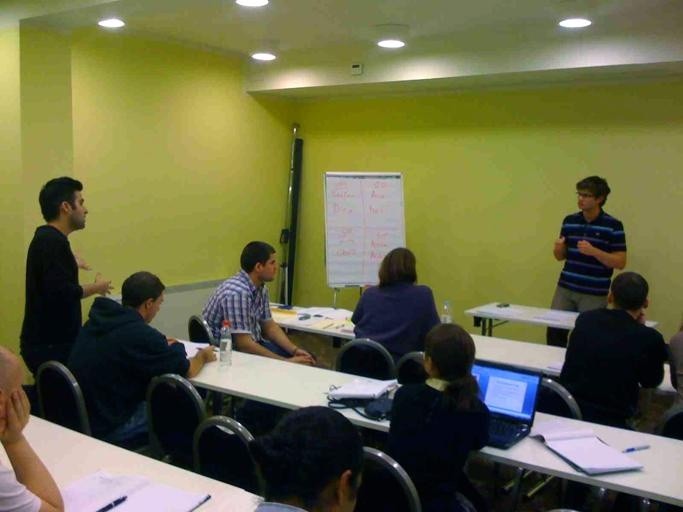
[344,284,360,288]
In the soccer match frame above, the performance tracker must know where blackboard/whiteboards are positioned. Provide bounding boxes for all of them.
[323,171,407,288]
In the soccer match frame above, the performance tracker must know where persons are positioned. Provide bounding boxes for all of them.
[0,345,64,511]
[250,404,364,512]
[351,248,442,360]
[350,323,491,510]
[17,177,115,375]
[200,239,315,432]
[657,320,681,436]
[65,267,218,441]
[556,273,667,432]
[544,174,627,346]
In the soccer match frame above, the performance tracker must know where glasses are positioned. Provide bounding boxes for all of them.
[575,191,594,198]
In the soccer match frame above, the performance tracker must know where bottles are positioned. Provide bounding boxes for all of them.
[219,321,231,369]
[441,301,450,324]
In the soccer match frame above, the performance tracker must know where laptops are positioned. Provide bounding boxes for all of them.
[470,359,542,449]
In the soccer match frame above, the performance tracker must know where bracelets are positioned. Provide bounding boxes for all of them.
[290,344,300,357]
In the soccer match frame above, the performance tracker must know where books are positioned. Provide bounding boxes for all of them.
[298,307,353,322]
[529,420,644,478]
[57,469,211,512]
[328,379,406,400]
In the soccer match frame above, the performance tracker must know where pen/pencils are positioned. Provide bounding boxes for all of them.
[622,444,651,454]
[96,495,128,512]
[196,346,219,353]
